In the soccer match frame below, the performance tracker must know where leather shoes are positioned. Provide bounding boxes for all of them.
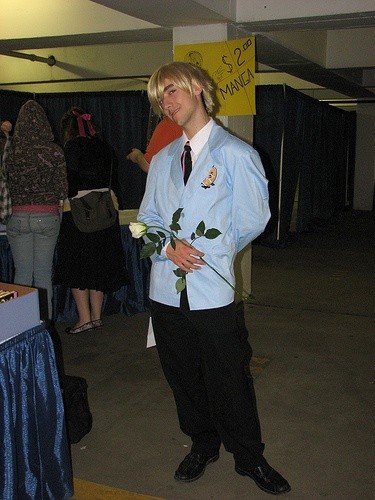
[235,457,291,495]
[173,451,219,482]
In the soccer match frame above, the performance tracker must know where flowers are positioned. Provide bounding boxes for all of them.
[129,208,247,304]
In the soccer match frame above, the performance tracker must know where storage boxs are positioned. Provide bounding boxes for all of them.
[0,282,40,343]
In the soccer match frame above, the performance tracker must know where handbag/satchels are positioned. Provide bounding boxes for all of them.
[69,190,116,232]
[0,140,13,225]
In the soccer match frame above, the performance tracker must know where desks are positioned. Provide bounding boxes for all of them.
[0,321,74,500]
[0,210,150,324]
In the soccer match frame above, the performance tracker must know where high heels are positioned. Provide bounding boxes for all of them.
[64,321,94,335]
[91,319,104,328]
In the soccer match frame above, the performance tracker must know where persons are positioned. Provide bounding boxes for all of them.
[53,107,130,334]
[136,61,290,494]
[0,101,68,328]
[127,116,183,172]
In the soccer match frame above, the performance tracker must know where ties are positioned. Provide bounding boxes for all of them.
[181,145,193,186]
